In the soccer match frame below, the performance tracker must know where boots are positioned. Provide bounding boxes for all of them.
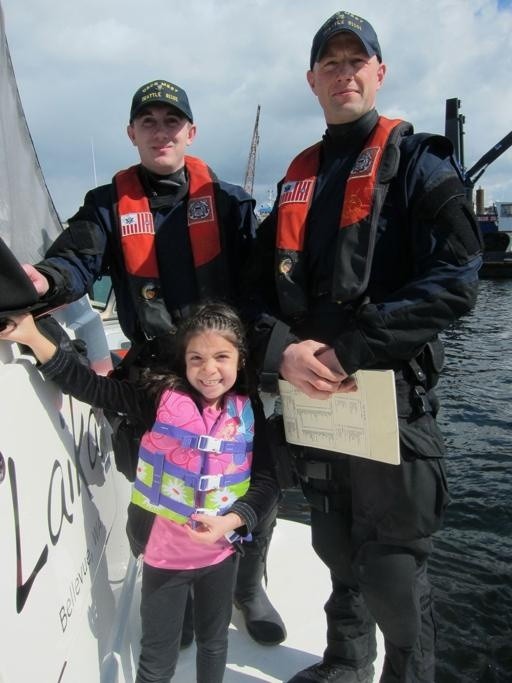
[233,556,288,648]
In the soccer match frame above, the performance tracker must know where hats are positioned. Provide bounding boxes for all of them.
[130,80,195,122]
[310,11,381,63]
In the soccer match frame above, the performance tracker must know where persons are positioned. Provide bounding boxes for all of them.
[242,6,485,682]
[18,80,288,649]
[0,300,282,682]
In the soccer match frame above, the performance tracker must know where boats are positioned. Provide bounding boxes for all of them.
[1,23,386,680]
[445,99,512,276]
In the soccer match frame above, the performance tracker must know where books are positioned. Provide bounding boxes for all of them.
[276,367,402,470]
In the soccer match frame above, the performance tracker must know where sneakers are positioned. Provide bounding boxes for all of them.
[285,658,378,683]
